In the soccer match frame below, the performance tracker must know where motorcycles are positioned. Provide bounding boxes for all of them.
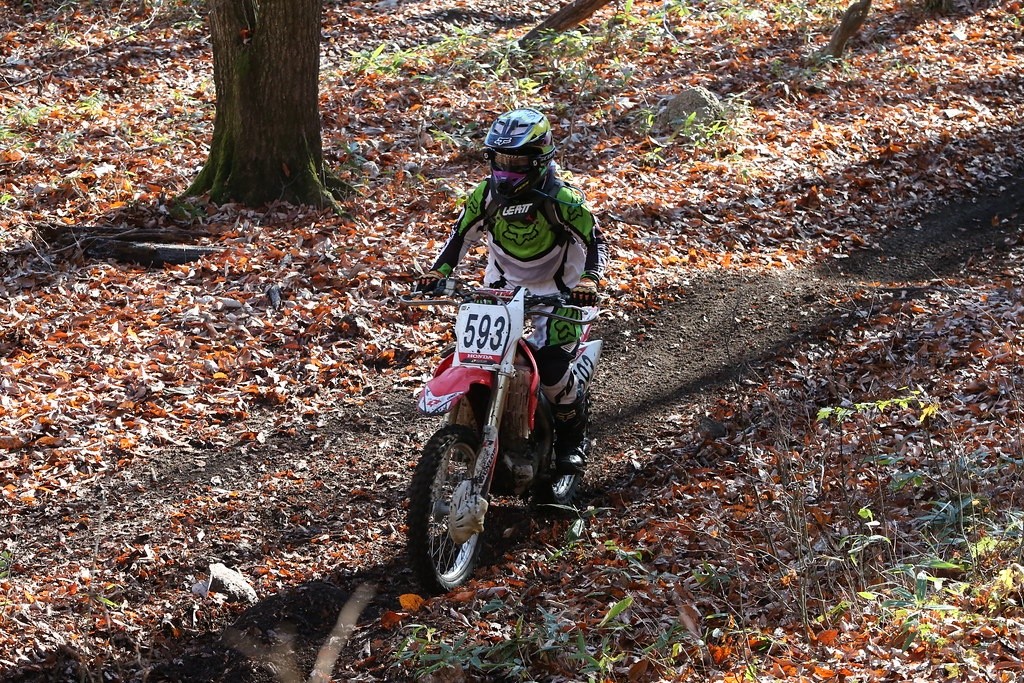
[398,271,617,595]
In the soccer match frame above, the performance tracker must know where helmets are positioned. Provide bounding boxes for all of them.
[483,107,556,206]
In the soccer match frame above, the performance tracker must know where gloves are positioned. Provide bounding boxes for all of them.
[414,270,444,294]
[570,277,600,307]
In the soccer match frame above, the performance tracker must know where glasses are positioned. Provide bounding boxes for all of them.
[486,149,537,172]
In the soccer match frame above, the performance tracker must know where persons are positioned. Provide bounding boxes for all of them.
[416,108,609,476]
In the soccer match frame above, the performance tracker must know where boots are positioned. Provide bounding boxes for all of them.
[551,395,586,475]
